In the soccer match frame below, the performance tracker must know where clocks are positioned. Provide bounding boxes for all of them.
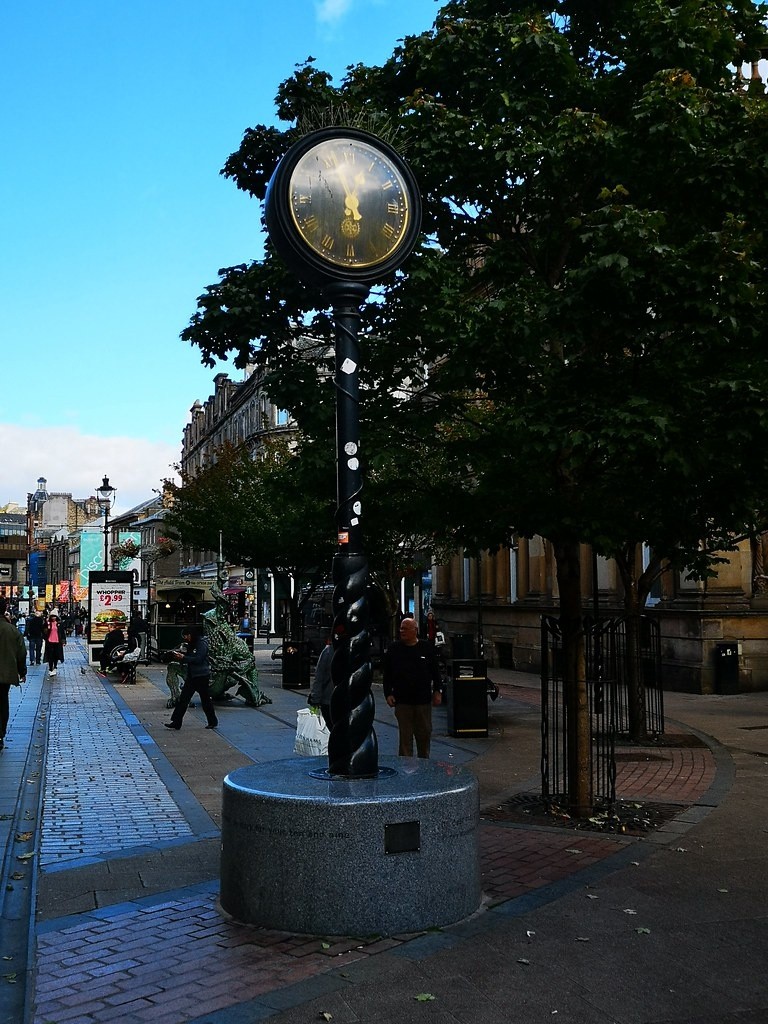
[288,138,412,270]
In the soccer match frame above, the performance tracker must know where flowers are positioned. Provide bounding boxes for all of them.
[121,540,141,552]
[156,536,173,549]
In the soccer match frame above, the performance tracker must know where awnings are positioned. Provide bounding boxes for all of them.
[222,588,247,595]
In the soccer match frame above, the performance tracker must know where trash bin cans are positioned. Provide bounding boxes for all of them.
[445,659,488,738]
[236,632,254,656]
[282,641,310,690]
[713,639,739,695]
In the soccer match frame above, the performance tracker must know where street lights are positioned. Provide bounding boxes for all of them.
[96,474,117,572]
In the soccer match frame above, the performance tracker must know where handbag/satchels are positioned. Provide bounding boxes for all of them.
[293,708,330,757]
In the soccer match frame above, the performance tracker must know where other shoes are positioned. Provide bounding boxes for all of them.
[165,722,181,730]
[49,668,57,677]
[96,669,106,678]
[205,724,214,729]
[30,661,34,665]
[36,663,41,665]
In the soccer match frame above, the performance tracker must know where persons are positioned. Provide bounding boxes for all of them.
[5,606,87,677]
[423,612,439,647]
[164,625,219,730]
[0,595,28,750]
[135,611,144,624]
[383,618,443,758]
[97,623,142,683]
[307,644,335,732]
[239,613,252,633]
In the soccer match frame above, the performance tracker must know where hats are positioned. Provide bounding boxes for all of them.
[49,608,59,617]
[35,611,41,617]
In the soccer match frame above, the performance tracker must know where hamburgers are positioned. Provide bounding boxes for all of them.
[94,609,126,631]
